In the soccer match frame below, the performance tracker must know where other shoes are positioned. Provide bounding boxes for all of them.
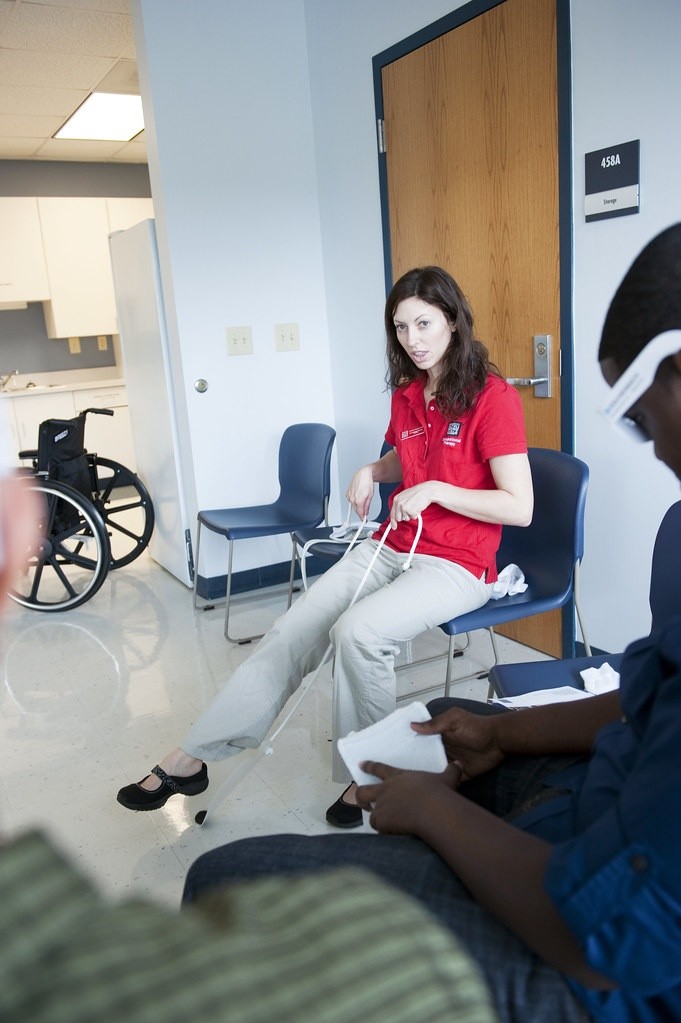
[117,761,208,813]
[326,781,363,828]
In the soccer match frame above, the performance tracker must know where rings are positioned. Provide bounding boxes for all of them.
[402,515,410,520]
[454,760,463,770]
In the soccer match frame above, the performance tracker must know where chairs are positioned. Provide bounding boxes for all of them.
[486,500,681,708]
[193,423,336,644]
[288,427,471,674]
[392,447,593,717]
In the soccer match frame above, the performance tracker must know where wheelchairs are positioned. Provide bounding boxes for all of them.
[0,407,156,613]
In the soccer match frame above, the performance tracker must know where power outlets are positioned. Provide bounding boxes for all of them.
[274,323,301,352]
[226,326,254,355]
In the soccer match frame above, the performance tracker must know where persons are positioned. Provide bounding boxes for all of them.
[117,266,533,829]
[181,220,681,1023]
[0,466,499,1023]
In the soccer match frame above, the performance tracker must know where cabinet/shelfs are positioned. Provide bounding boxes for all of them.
[73,378,138,479]
[37,196,155,338]
[0,197,51,311]
[0,385,76,495]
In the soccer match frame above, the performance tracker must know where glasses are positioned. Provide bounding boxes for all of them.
[597,329,681,442]
